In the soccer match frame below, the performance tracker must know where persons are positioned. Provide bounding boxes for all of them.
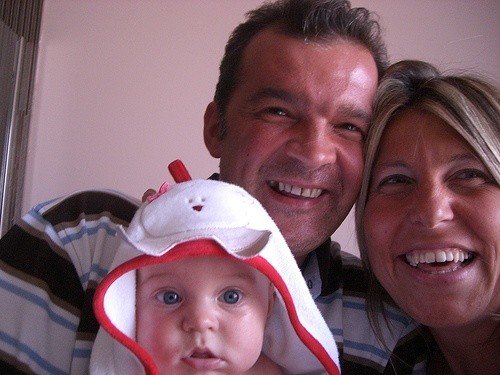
[87,159,343,375]
[358,59,500,375]
[0,0,429,375]
